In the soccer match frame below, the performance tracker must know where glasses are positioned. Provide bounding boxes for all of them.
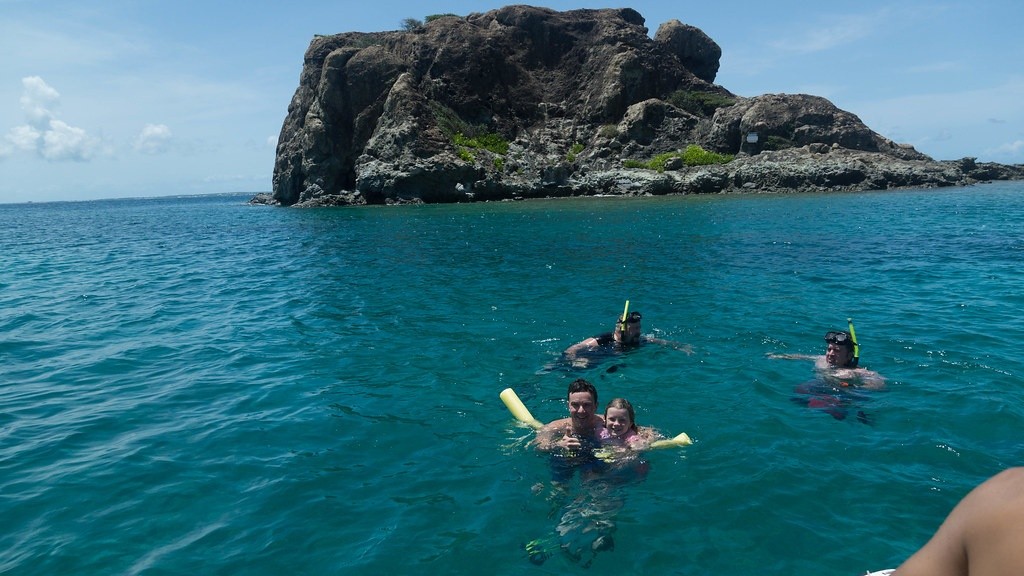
[631,312,641,319]
[825,331,847,341]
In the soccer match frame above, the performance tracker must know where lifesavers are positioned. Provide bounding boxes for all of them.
[498,386,691,453]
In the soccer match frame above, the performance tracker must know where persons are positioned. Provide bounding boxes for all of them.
[595,398,636,436]
[550,379,605,429]
[584,311,642,348]
[825,329,853,367]
[890,467,1024,576]
